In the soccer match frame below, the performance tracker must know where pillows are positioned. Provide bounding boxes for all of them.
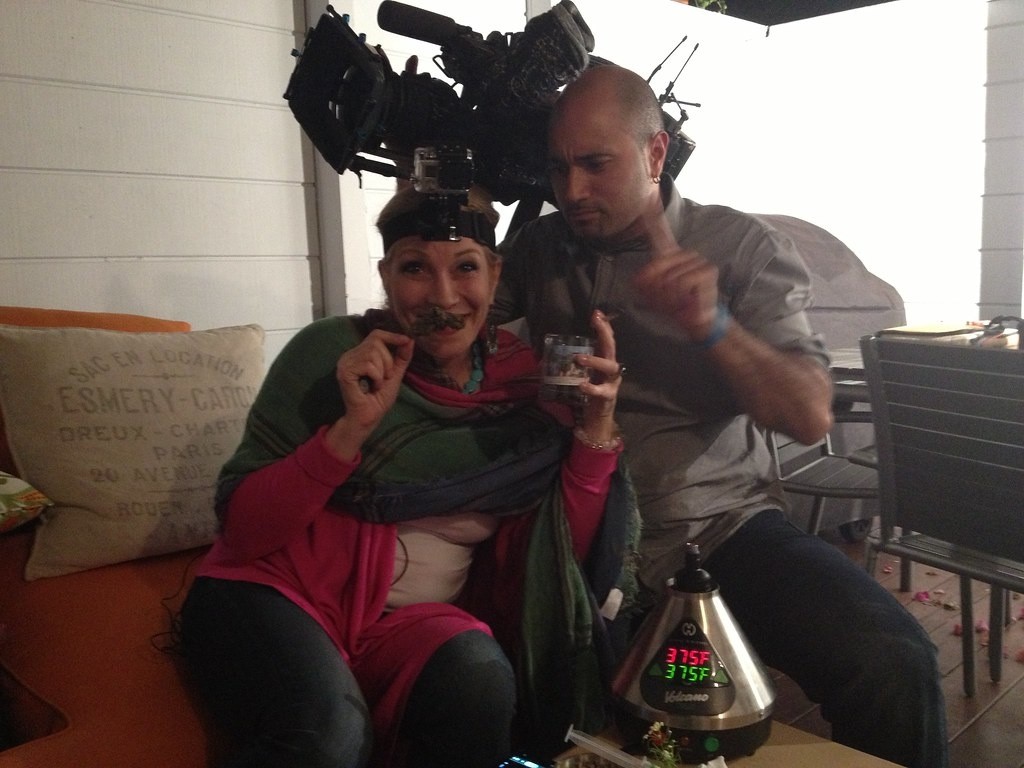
[0,323,266,582]
[0,471,55,534]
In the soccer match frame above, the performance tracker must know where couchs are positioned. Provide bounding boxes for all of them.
[0,306,216,768]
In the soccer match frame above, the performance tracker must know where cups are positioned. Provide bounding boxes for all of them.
[538,333,596,406]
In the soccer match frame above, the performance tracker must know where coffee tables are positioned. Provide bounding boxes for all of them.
[555,720,909,768]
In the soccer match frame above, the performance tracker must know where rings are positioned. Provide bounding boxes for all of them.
[608,364,629,381]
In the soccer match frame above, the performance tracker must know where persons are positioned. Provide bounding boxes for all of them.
[487,63,952,767]
[175,182,643,768]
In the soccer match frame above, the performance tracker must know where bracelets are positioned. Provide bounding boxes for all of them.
[688,306,728,350]
[573,420,623,449]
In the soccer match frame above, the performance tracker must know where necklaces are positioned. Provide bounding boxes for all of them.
[461,341,484,394]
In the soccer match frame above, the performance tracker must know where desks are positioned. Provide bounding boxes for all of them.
[828,348,866,381]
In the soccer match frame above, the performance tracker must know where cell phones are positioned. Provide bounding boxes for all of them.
[498,753,558,768]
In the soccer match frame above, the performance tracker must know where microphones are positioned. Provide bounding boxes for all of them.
[376,0,483,48]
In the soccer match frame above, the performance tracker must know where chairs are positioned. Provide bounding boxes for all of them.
[859,335,1024,698]
[766,430,881,535]
